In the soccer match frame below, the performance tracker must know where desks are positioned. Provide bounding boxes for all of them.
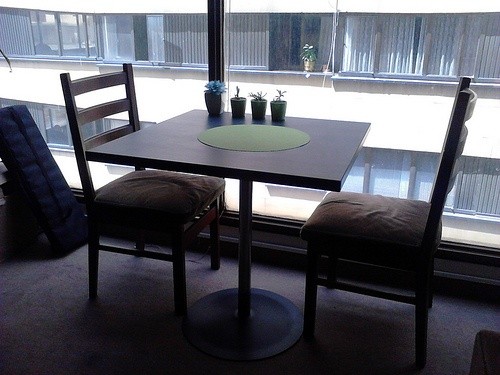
[86,110,373,362]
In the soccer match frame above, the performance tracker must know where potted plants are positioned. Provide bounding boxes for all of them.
[270,89,289,122]
[298,43,316,72]
[230,85,246,120]
[249,90,268,121]
[203,80,225,117]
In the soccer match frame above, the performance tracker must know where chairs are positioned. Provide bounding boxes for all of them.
[58,64,226,318]
[297,77,472,375]
